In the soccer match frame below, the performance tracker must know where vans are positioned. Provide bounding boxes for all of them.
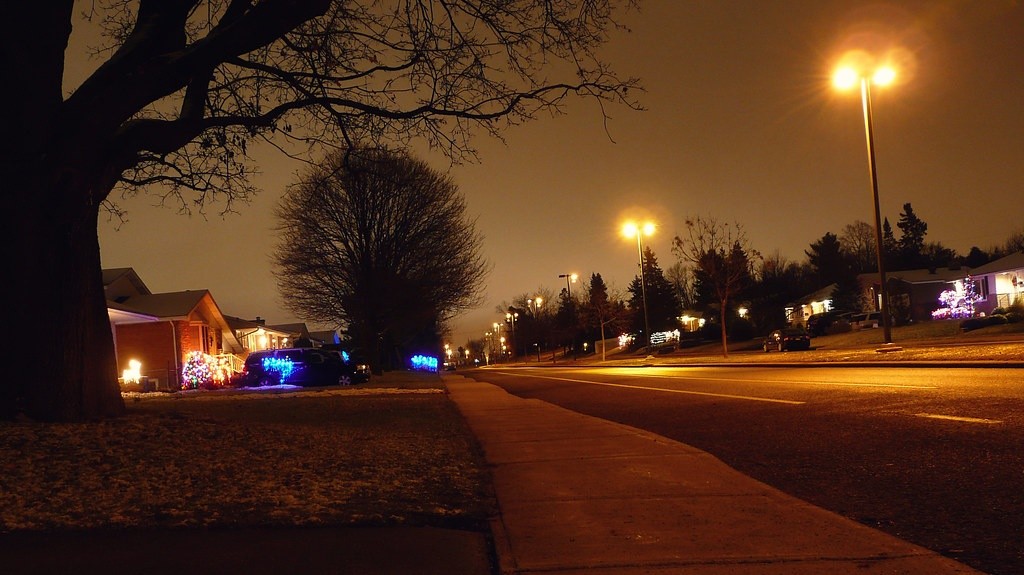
[247,346,371,389]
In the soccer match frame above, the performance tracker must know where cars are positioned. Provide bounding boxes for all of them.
[763,328,811,351]
[445,362,457,371]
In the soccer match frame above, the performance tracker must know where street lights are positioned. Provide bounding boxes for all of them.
[621,217,659,349]
[559,274,572,298]
[506,312,518,341]
[830,49,904,352]
[527,297,543,318]
